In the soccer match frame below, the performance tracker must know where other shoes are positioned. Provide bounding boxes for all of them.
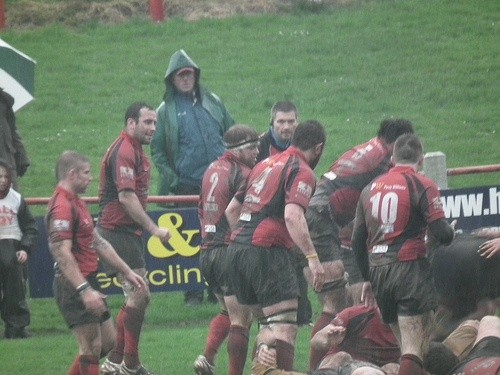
[187,298,201,307]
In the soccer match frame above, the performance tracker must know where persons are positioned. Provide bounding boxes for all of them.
[224,120,326,375]
[0,87,30,191]
[351,135,454,375]
[251,303,401,375]
[95,102,172,375]
[150,49,237,307]
[422,219,500,375]
[0,158,39,339]
[194,123,261,375]
[253,101,315,328]
[44,152,147,375]
[339,218,365,307]
[304,119,414,375]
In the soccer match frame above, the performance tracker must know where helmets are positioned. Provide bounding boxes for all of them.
[222,125,258,150]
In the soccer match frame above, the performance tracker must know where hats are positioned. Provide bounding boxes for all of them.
[177,67,194,76]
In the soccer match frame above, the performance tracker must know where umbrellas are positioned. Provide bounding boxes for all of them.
[0,38,37,114]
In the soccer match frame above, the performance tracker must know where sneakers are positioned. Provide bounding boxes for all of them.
[194,355,215,375]
[119,360,154,375]
[101,358,120,375]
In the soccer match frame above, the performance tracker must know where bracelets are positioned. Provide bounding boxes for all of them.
[75,281,92,297]
[306,254,318,259]
[257,341,268,351]
